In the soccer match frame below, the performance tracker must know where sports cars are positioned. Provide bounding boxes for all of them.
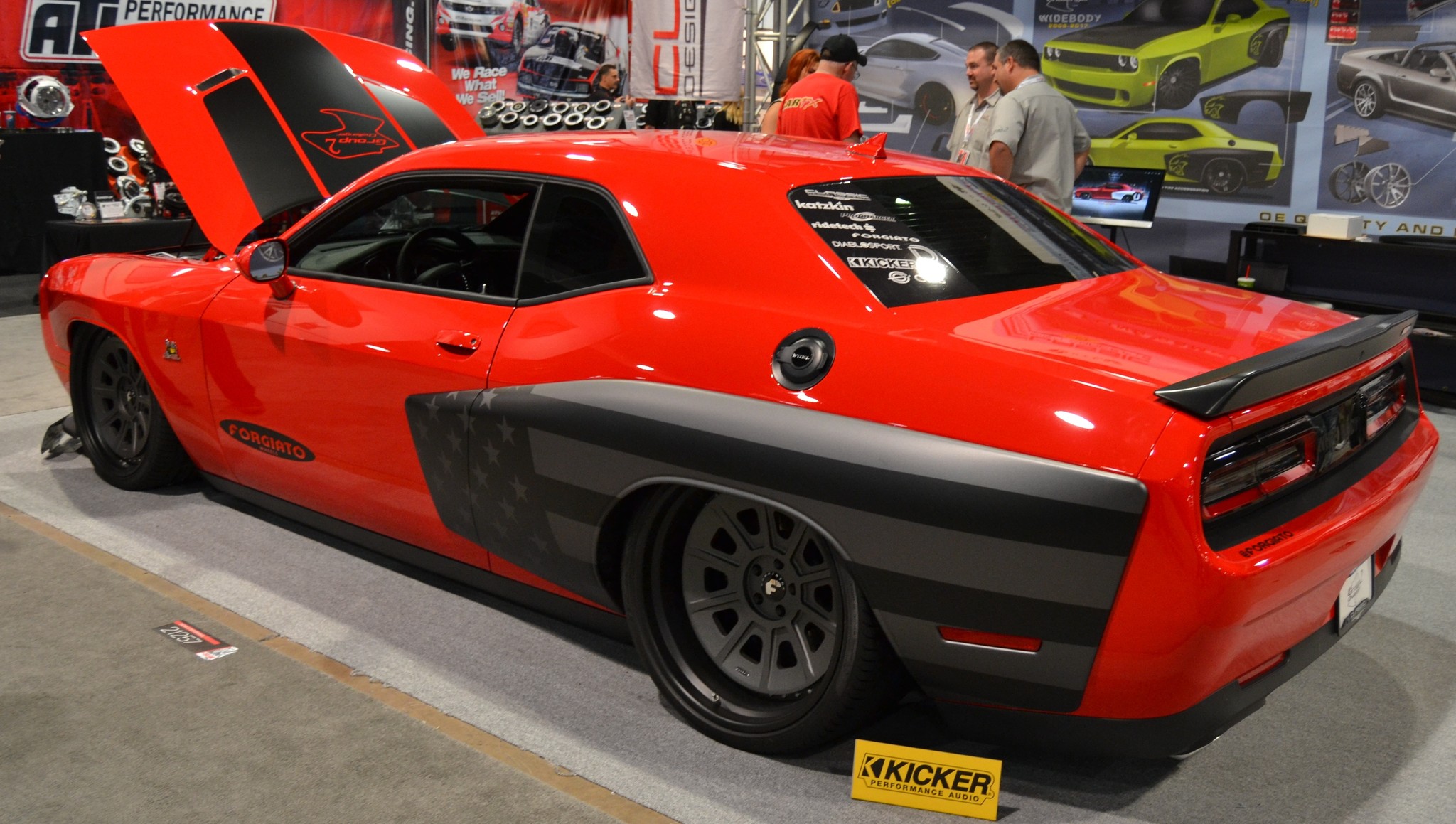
[434,1,553,53]
[519,21,626,105]
[1042,1,1290,108]
[1087,114,1278,191]
[40,14,1438,768]
[807,2,1019,122]
[1337,38,1455,132]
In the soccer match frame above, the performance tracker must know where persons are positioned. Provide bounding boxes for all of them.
[760,47,821,135]
[776,32,867,145]
[644,100,697,131]
[946,41,1004,173]
[713,84,744,133]
[987,39,1091,218]
[582,64,637,109]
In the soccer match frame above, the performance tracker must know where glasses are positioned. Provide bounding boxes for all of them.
[843,63,861,80]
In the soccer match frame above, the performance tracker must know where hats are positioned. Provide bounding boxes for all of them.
[819,34,868,67]
